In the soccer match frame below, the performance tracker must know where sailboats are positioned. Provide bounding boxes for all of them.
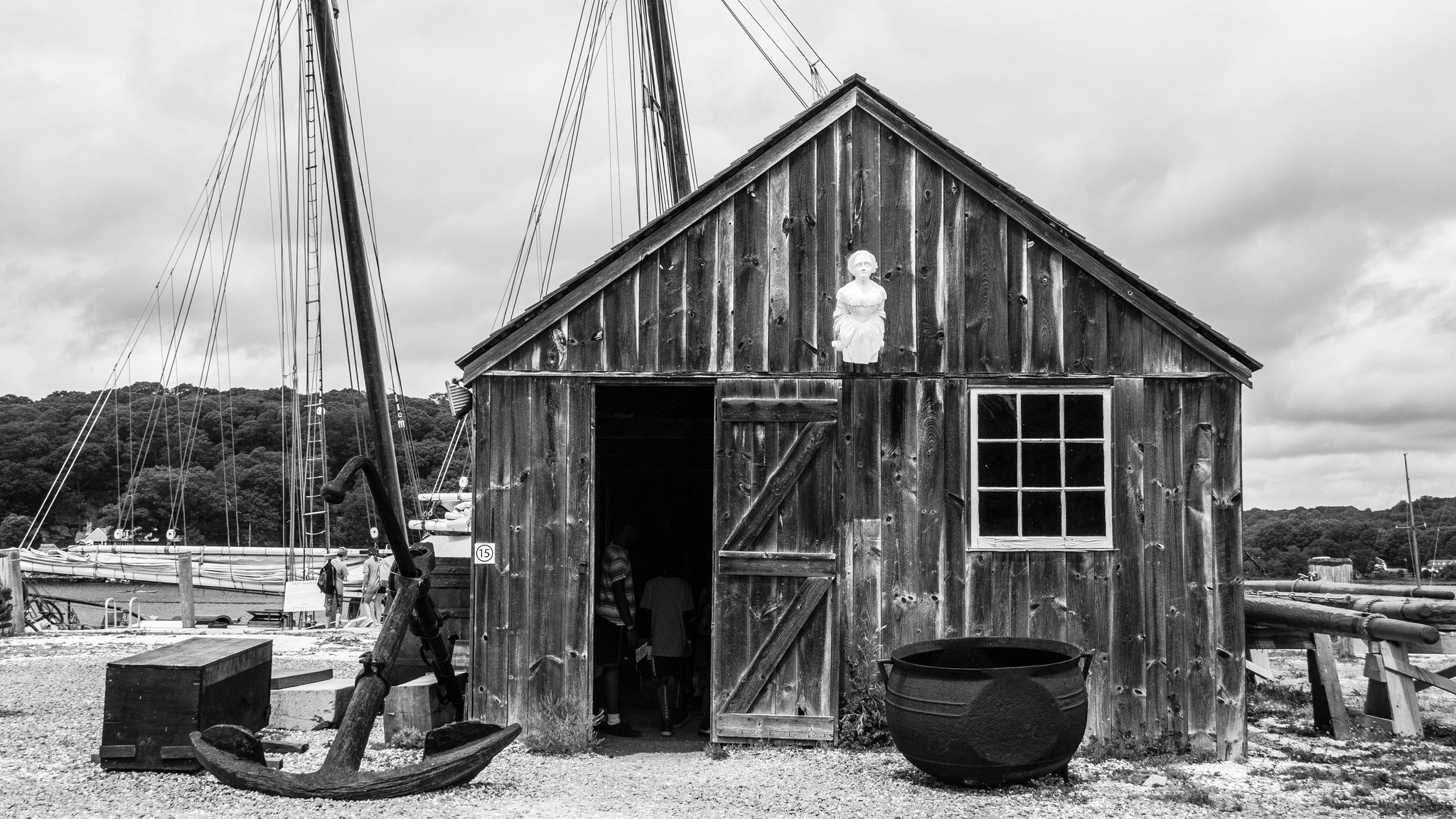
[1,0,844,630]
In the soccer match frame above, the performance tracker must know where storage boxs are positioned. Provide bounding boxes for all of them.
[385,669,467,740]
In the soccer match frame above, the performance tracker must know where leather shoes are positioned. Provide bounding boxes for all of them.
[596,718,607,732]
[604,722,641,738]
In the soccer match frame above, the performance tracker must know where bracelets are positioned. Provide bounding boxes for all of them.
[626,624,635,630]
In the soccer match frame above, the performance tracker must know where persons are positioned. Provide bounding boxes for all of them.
[637,559,697,738]
[362,547,385,627]
[324,546,349,628]
[831,249,888,364]
[592,520,649,738]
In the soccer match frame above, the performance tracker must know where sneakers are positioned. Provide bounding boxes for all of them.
[659,721,673,735]
[674,710,690,727]
[698,726,711,737]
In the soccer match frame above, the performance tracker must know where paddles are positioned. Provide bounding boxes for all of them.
[133,585,144,593]
